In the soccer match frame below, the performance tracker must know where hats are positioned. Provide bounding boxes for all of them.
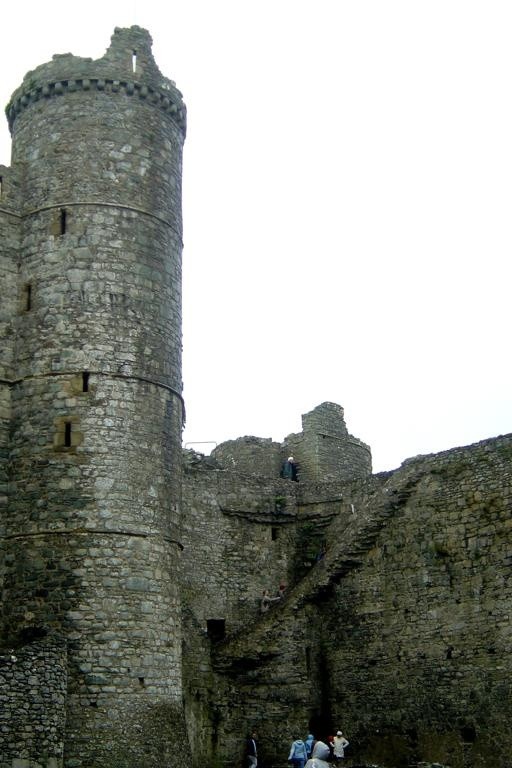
[337,730,342,736]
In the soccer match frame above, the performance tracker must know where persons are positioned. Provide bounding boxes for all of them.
[288,731,349,768]
[261,585,286,612]
[283,457,294,481]
[246,733,258,768]
